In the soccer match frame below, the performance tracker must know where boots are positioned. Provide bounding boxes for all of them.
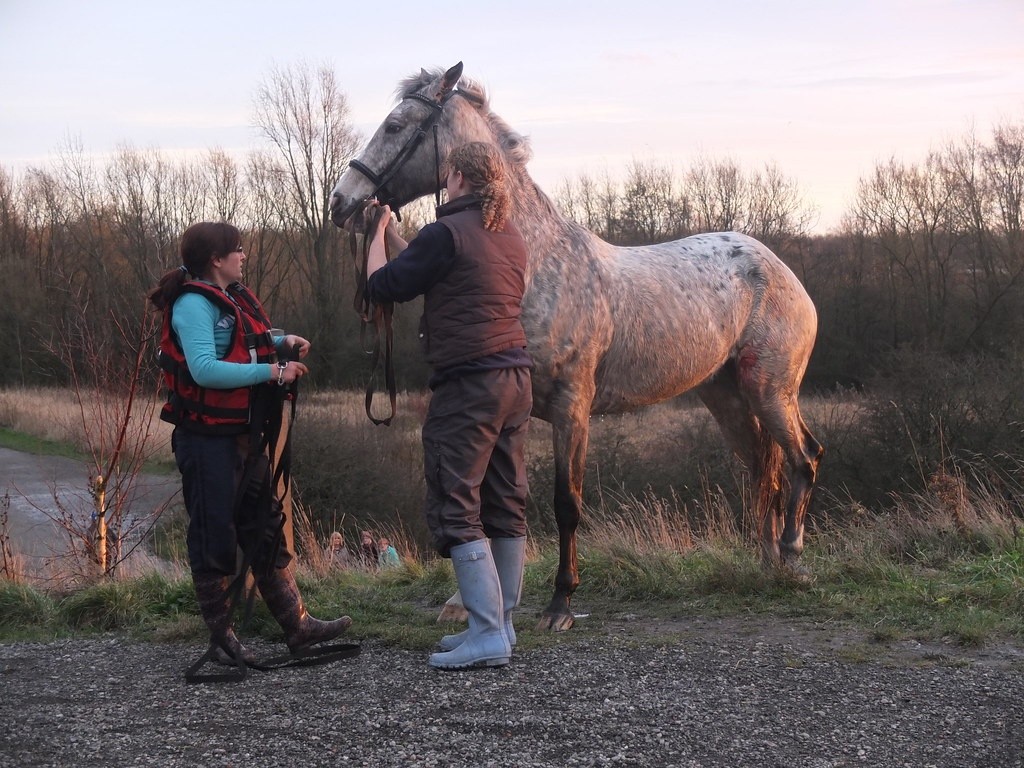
[440,535,527,652]
[252,565,352,655]
[428,538,511,667]
[194,576,255,662]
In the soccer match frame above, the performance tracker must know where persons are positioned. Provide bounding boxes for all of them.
[146,221,359,673]
[365,140,534,669]
[356,530,400,574]
[326,532,349,574]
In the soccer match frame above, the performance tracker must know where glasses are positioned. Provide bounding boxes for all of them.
[231,247,243,254]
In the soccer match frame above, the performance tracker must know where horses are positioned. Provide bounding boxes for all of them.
[324,61,827,635]
